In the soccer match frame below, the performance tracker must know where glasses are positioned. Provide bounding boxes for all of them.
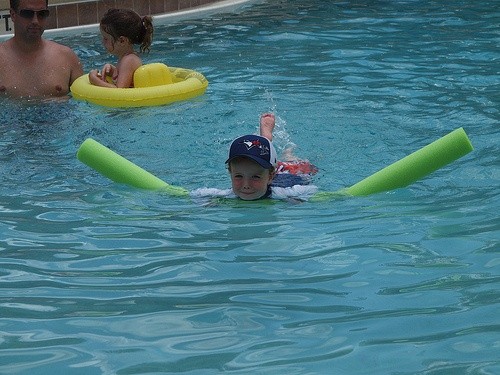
[15,8,49,18]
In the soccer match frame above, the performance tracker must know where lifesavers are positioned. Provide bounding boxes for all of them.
[69,62,208,107]
[76,137,190,197]
[310,126,474,202]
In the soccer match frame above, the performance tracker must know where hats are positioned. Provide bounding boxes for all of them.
[225,134,277,172]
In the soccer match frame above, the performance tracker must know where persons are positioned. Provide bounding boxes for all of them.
[88,8,154,88]
[0,0,85,105]
[191,112,321,201]
